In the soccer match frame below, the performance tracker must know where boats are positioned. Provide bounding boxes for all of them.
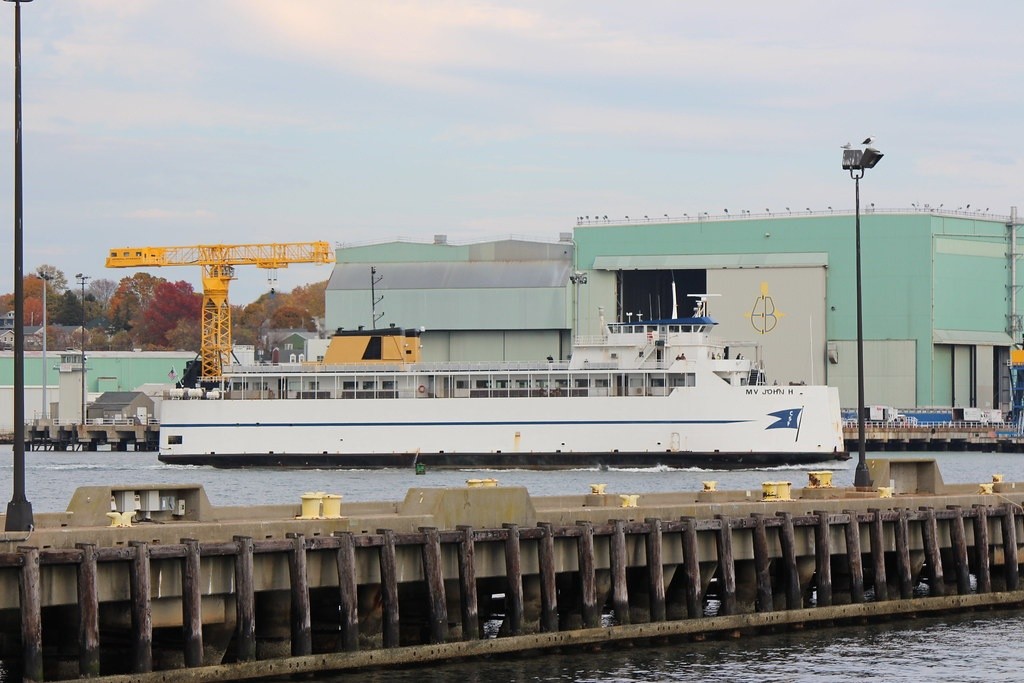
[157,294,850,476]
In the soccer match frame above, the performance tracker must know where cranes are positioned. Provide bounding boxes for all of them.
[106,240,337,390]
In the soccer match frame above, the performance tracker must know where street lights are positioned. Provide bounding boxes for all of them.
[36,271,55,421]
[76,273,90,425]
[841,149,884,491]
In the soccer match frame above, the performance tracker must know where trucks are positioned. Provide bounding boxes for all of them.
[864,405,894,424]
[952,407,987,426]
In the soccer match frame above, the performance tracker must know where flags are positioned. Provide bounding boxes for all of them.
[168,367,176,380]
